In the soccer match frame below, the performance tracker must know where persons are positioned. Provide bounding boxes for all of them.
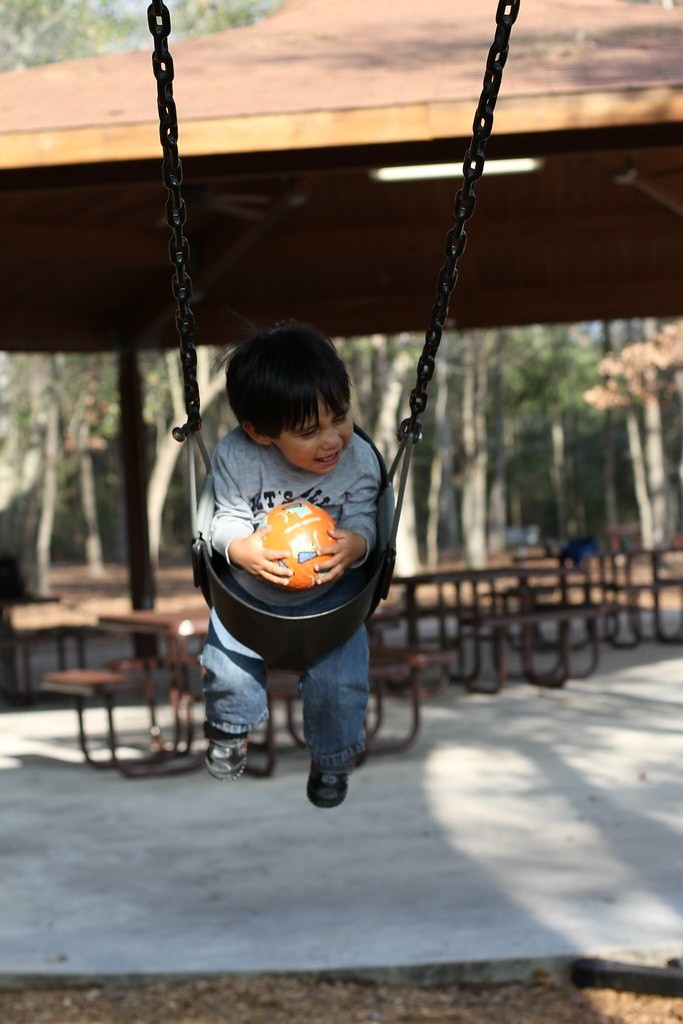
[196,323,396,807]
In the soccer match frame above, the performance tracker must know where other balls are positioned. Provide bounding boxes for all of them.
[252,500,343,593]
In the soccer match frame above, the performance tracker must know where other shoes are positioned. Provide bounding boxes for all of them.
[307,761,349,808]
[204,737,248,781]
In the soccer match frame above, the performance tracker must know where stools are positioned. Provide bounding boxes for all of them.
[0,540,682,779]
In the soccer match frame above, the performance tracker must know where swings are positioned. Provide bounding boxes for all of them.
[142,0,526,676]
